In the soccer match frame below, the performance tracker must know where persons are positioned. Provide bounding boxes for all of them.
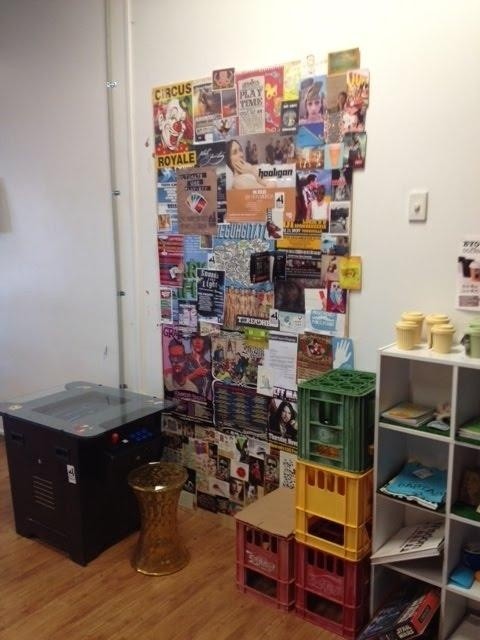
[163,88,362,513]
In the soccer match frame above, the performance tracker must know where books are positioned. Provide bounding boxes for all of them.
[370,518,446,565]
[458,414,480,444]
[383,400,435,427]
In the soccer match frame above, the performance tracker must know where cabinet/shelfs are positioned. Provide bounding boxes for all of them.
[369,340,480,640]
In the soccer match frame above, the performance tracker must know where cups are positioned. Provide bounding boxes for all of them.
[431,324,457,353]
[466,320,480,359]
[400,310,425,344]
[468,265,480,283]
[425,313,450,344]
[462,259,475,277]
[395,322,418,351]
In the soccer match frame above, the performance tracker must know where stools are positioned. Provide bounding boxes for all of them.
[127,461,190,577]
